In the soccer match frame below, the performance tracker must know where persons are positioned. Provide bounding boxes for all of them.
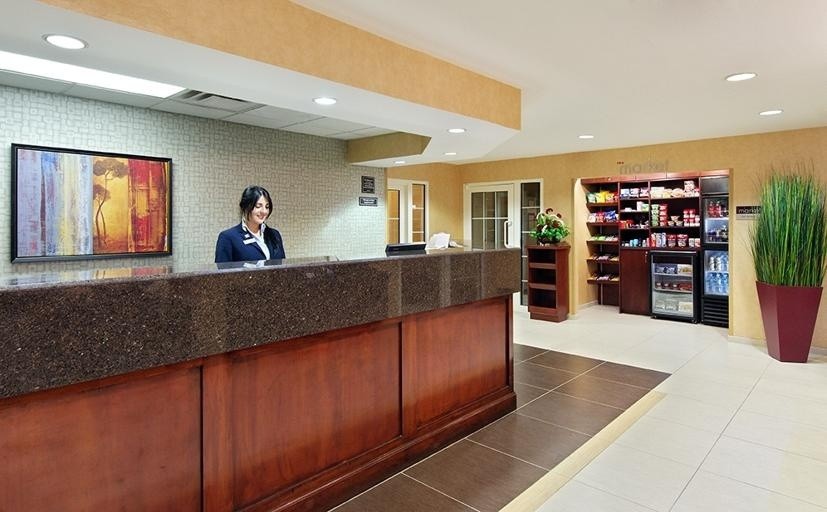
[214,185,286,264]
[218,258,282,271]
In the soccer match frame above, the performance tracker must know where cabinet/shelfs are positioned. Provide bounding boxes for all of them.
[581,168,702,316]
[527,245,570,322]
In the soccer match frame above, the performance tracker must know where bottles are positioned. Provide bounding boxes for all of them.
[704,198,728,295]
[621,237,650,248]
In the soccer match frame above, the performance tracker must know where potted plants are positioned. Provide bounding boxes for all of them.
[740,158,827,363]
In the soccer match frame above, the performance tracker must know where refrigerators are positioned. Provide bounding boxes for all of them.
[649,250,697,320]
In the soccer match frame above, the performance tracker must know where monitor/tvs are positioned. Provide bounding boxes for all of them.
[385,242,427,251]
[386,250,426,257]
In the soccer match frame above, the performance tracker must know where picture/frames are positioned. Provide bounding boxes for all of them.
[11,143,172,264]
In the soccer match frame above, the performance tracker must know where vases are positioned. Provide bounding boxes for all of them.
[537,235,560,244]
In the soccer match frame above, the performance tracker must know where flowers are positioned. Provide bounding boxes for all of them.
[528,208,570,242]
